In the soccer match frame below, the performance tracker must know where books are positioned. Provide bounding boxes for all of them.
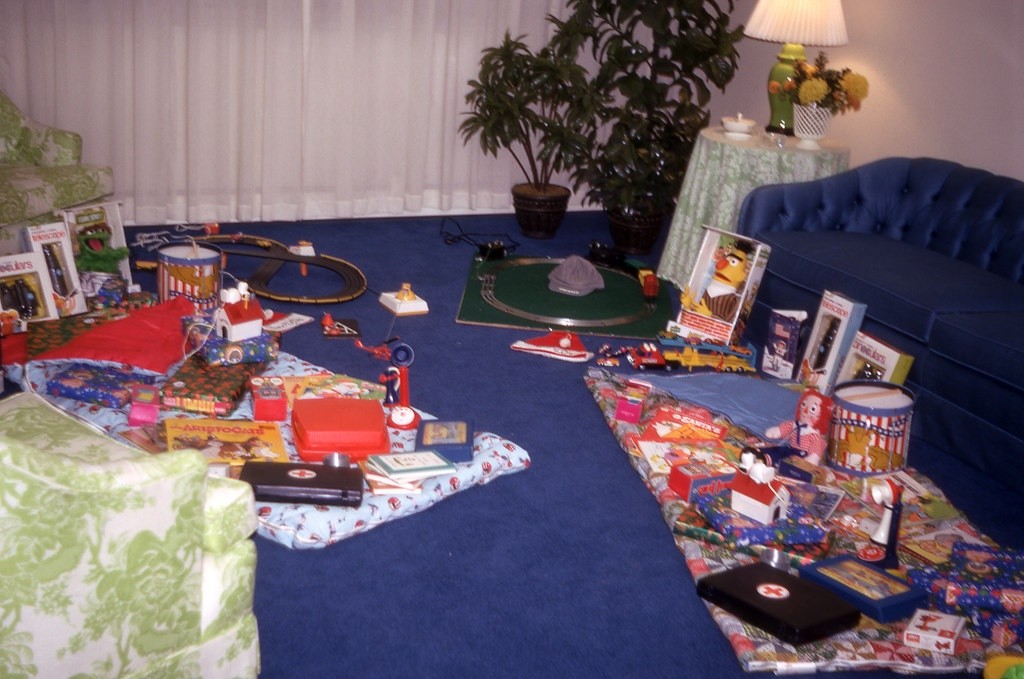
[119,416,290,468]
[630,405,733,475]
[357,450,458,494]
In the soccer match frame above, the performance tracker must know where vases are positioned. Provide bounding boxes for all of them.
[791,102,835,150]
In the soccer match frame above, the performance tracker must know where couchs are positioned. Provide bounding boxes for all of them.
[0,91,115,254]
[0,391,258,679]
[735,156,1024,552]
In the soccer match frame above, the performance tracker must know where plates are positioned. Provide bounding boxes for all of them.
[723,133,751,140]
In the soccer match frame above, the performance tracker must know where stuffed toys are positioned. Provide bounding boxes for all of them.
[739,387,832,468]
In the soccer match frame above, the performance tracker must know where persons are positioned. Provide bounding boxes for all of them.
[679,240,755,326]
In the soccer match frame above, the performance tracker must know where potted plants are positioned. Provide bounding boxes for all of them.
[457,20,603,238]
[551,0,746,243]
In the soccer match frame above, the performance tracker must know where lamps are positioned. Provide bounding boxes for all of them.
[743,0,849,135]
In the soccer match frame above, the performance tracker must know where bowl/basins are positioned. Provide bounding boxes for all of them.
[722,116,756,133]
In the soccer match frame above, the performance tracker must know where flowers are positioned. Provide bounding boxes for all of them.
[782,50,870,110]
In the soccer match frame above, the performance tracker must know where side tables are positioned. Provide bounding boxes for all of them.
[659,125,854,290]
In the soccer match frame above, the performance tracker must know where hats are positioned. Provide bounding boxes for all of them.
[547,254,605,296]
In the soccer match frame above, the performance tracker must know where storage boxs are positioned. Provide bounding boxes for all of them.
[797,290,869,395]
[760,305,808,381]
[24,220,89,317]
[61,202,138,305]
[0,252,61,323]
[677,224,771,342]
[165,313,478,473]
[834,332,916,396]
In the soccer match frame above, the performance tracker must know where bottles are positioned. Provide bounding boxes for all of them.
[0,279,38,318]
[42,243,69,297]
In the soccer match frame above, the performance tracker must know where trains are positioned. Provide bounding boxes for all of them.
[585,237,660,301]
